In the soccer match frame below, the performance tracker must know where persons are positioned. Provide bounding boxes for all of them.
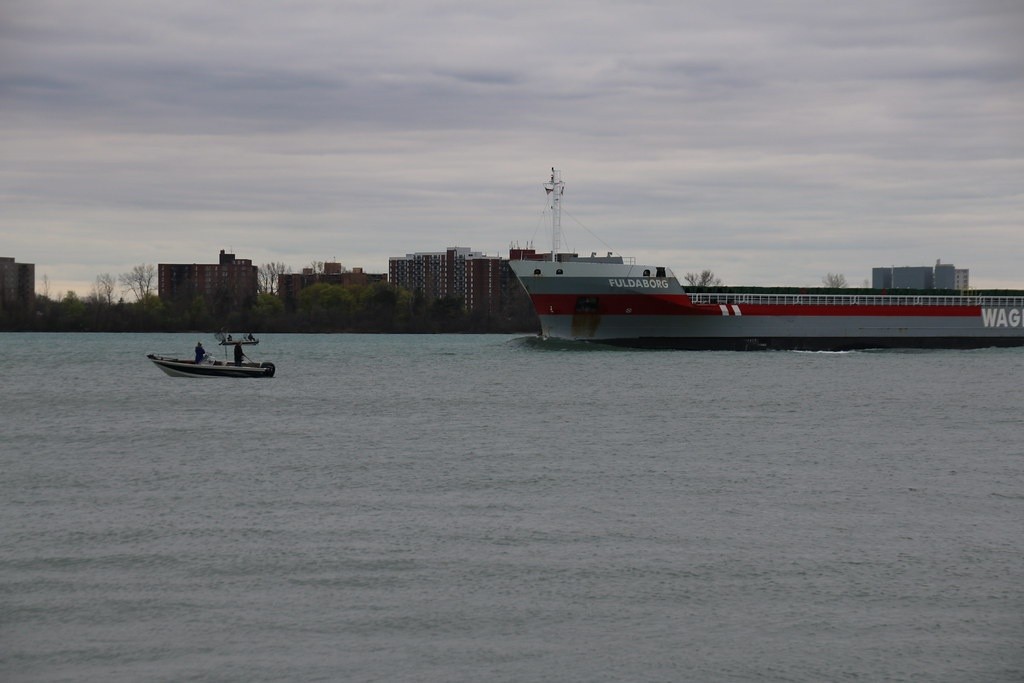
[227,335,232,341]
[195,341,205,364]
[234,340,245,367]
[248,333,255,341]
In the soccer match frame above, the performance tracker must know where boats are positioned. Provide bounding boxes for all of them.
[144,332,275,379]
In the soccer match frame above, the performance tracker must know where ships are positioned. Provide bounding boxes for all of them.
[505,167,1024,352]
